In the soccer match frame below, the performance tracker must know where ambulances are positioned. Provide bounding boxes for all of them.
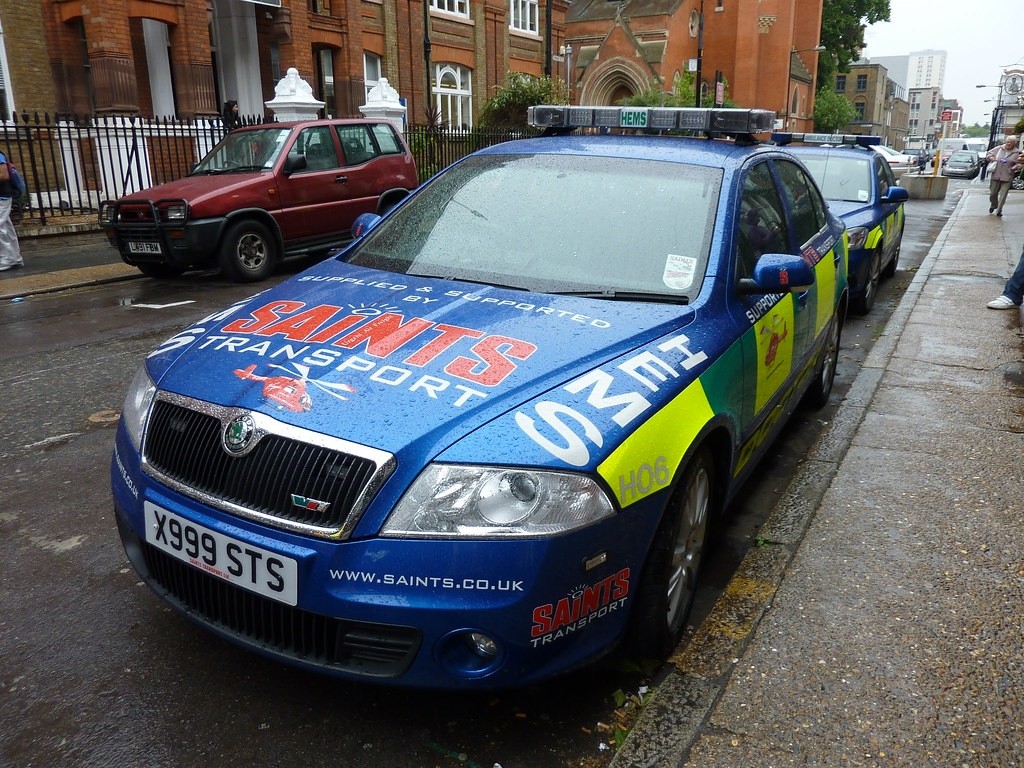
[767,129,909,316]
[110,107,850,695]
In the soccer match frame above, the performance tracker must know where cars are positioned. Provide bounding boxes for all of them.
[820,142,920,182]
[899,148,927,171]
[925,149,930,162]
[98,119,419,284]
[940,149,982,180]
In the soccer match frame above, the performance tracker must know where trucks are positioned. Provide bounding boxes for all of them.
[935,137,988,166]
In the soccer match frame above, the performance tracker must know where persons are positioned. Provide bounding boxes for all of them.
[0,150,24,271]
[986,135,1024,217]
[987,243,1024,309]
[1008,77,1019,92]
[222,100,245,163]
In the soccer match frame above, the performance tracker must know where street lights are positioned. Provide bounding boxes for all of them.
[975,83,1004,182]
[785,47,826,134]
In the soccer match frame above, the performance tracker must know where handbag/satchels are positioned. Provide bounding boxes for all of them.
[987,148,1002,172]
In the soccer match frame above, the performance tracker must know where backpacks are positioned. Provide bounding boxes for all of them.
[0,148,26,200]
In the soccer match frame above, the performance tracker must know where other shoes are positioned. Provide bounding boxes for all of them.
[989,208,992,212]
[998,214,1002,217]
[987,295,1021,309]
